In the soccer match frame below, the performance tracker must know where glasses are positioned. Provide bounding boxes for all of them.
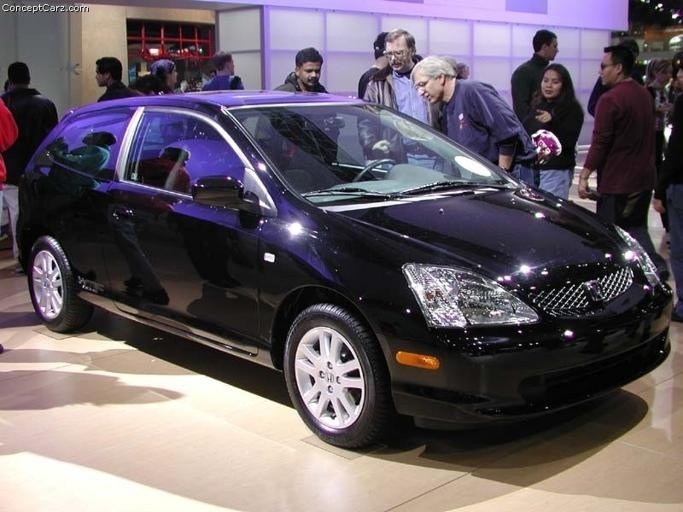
[383,48,408,57]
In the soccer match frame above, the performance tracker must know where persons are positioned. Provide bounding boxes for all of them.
[1,62,59,272]
[30,131,117,243]
[0,98,18,354]
[254,50,338,168]
[4,81,12,93]
[180,211,243,292]
[94,52,249,144]
[111,147,189,307]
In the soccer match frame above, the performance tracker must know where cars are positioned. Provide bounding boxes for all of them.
[13,87,675,453]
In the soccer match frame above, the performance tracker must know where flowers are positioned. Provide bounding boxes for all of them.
[528,127,563,165]
